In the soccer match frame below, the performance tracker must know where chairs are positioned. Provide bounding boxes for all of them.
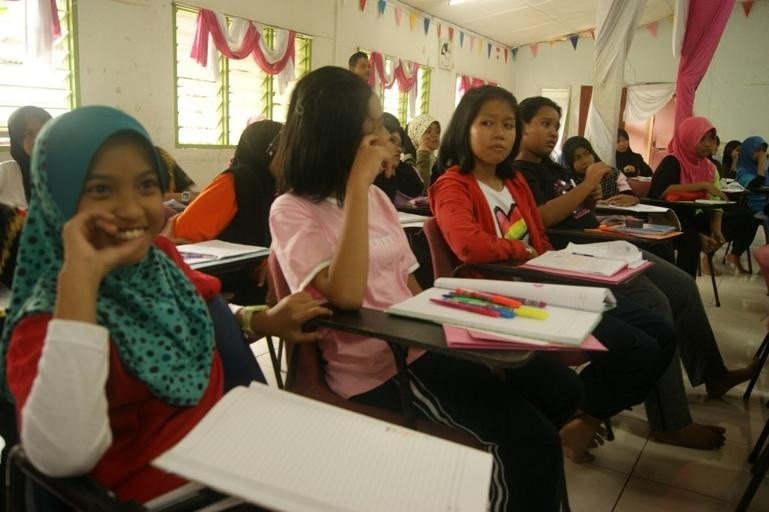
[0,175,768,512]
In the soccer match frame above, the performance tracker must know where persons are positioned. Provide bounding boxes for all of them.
[426,85,680,463]
[704,136,769,277]
[349,53,371,82]
[615,129,653,176]
[513,96,763,451]
[0,106,333,512]
[374,113,442,213]
[173,118,285,248]
[563,139,640,206]
[0,106,53,211]
[648,117,727,279]
[268,67,583,512]
[156,146,199,205]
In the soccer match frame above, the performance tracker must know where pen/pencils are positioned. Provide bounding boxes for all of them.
[572,252,594,257]
[429,288,549,320]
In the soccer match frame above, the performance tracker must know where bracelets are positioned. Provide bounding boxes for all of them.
[241,305,269,339]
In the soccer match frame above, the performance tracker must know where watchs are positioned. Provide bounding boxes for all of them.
[182,191,190,204]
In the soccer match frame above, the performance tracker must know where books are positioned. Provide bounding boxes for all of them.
[526,242,643,276]
[175,240,269,264]
[385,277,617,344]
[610,203,682,231]
